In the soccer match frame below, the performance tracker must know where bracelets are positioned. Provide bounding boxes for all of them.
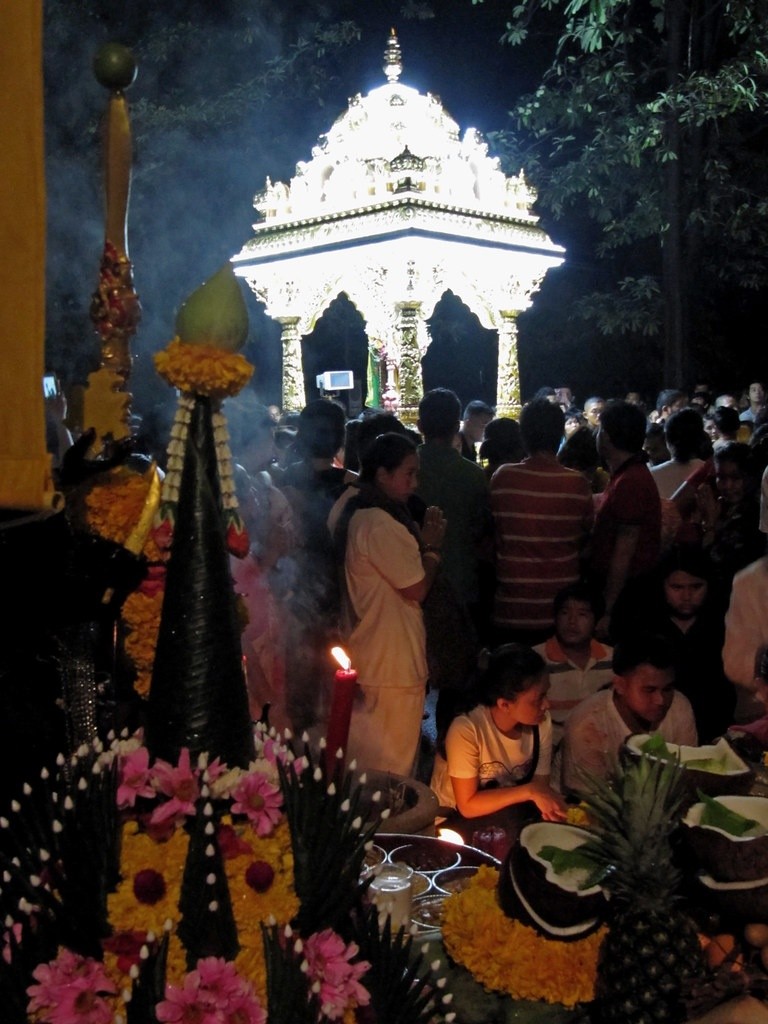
[419,545,443,559]
[422,552,439,565]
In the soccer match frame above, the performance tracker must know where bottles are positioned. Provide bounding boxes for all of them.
[366,862,413,942]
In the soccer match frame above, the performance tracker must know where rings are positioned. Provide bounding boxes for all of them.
[433,523,437,525]
[427,522,431,524]
[440,525,442,528]
[424,521,427,523]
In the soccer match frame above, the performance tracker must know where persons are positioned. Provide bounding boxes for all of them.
[562,624,698,804]
[41,381,768,761]
[526,584,614,760]
[619,546,736,743]
[326,431,447,777]
[429,643,576,826]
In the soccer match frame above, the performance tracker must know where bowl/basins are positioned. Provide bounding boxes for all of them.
[358,843,480,930]
